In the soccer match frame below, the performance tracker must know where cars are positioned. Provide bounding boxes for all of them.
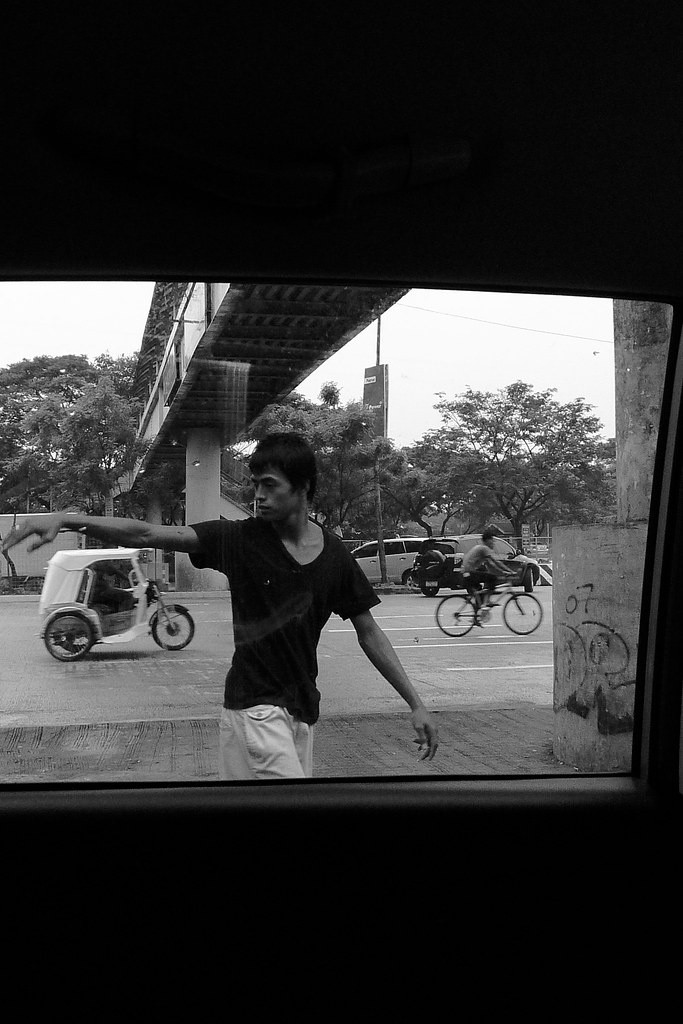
[351,537,430,594]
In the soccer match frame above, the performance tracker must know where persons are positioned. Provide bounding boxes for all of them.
[461,533,519,607]
[0,432,438,780]
[98,568,134,613]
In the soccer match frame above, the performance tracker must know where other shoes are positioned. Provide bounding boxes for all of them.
[486,603,499,608]
[476,623,484,628]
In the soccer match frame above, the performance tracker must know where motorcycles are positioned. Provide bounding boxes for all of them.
[37,545,196,663]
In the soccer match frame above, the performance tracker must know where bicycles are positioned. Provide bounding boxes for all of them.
[436,572,544,637]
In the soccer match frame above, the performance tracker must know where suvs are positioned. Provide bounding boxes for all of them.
[413,533,540,597]
